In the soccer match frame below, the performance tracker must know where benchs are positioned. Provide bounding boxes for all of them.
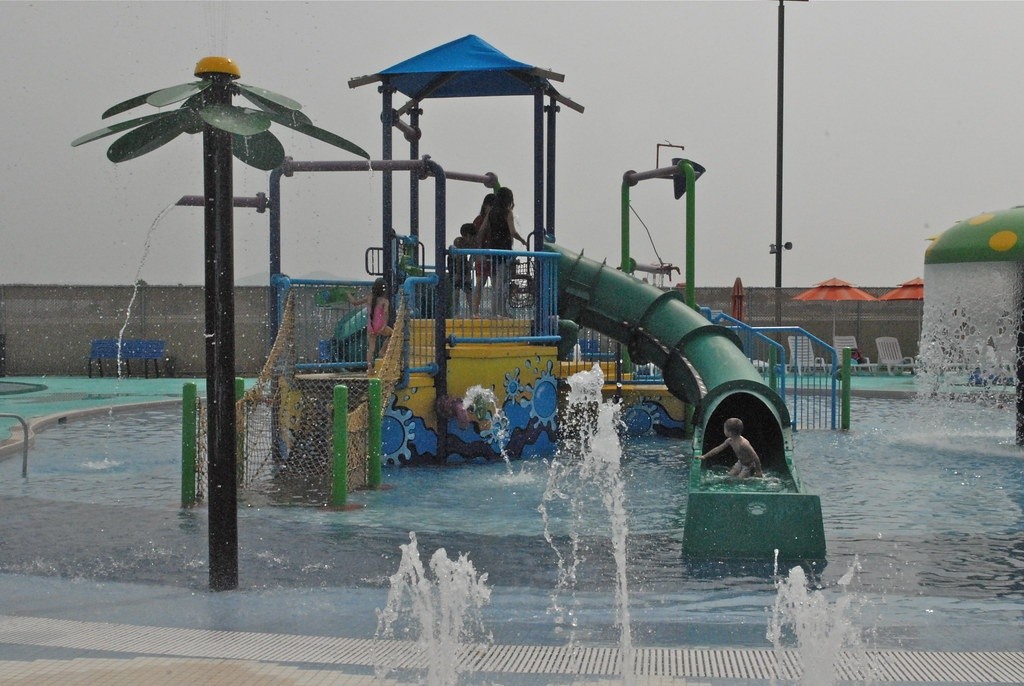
[87,339,165,378]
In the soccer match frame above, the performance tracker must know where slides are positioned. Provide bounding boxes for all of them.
[540,243,806,494]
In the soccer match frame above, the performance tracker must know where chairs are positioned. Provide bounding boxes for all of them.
[875,336,914,376]
[788,336,826,376]
[834,335,872,375]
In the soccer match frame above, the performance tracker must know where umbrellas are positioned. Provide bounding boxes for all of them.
[792,279,877,347]
[731,279,746,326]
[882,276,924,344]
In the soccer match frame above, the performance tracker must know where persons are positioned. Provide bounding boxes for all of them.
[448,187,528,321]
[695,417,764,478]
[851,348,868,366]
[346,276,391,380]
